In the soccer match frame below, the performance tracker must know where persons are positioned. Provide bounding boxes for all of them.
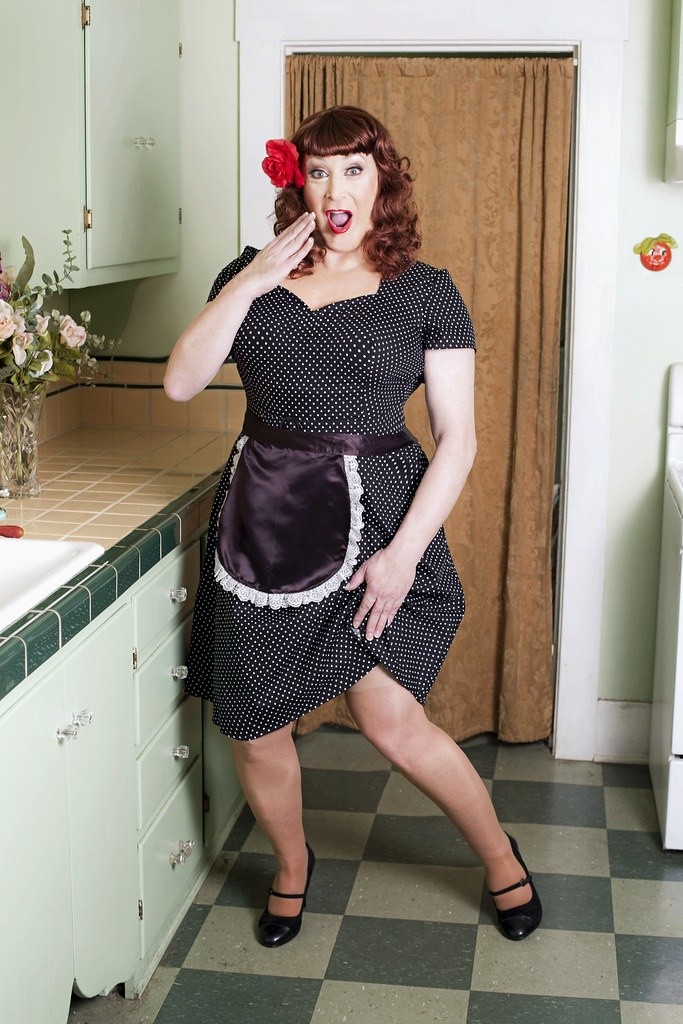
[162,105,544,947]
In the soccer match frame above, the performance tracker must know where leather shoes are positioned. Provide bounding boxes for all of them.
[488,829,543,939]
[256,843,314,947]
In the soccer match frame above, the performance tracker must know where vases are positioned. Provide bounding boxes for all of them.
[0,387,46,498]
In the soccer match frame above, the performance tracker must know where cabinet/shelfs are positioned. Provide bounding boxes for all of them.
[0,517,247,1024]
[0,0,182,296]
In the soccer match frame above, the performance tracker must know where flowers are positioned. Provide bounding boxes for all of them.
[261,139,306,189]
[0,229,122,486]
